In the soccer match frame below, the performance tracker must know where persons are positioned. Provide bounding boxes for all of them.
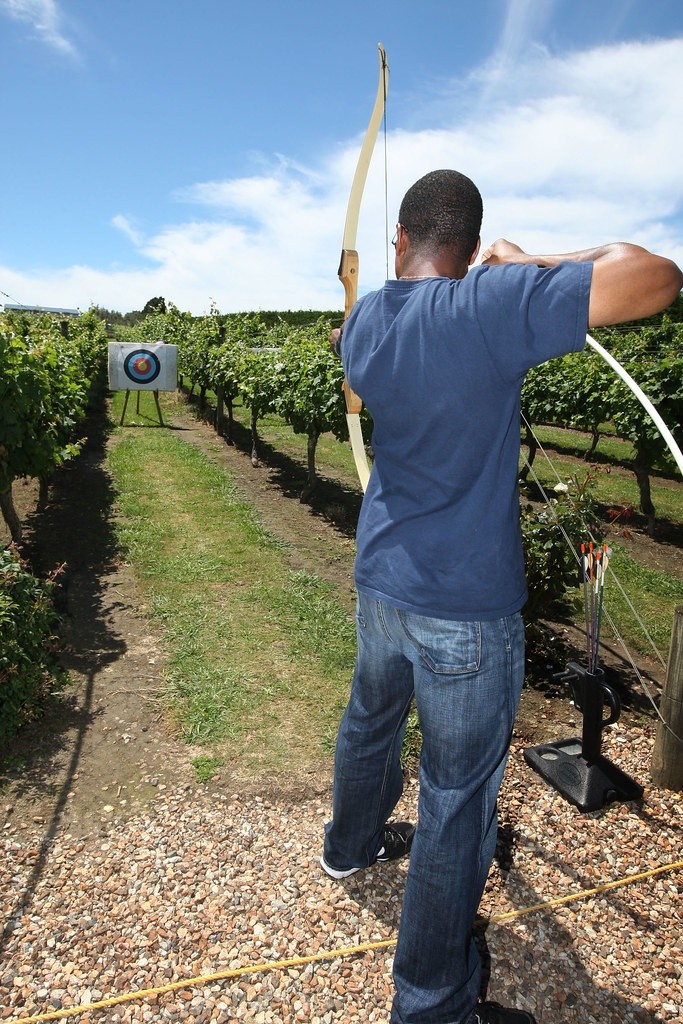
[319,169,682,1024]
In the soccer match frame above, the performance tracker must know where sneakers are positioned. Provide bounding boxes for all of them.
[467,1000,535,1024]
[319,822,416,879]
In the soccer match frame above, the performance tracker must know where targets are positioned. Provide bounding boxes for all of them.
[123,348,161,385]
